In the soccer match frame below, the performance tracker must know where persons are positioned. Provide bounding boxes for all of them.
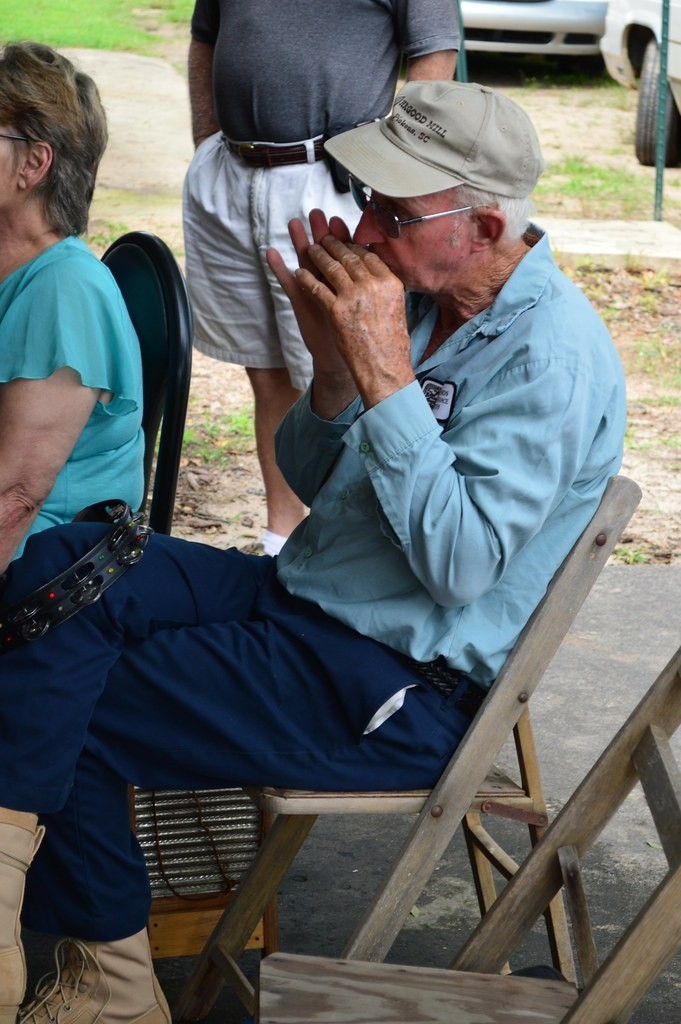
[0,79,625,1024]
[0,42,145,579]
[186,0,463,557]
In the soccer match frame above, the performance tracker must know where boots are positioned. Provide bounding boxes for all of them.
[0,807,47,1024]
[19,926,172,1023]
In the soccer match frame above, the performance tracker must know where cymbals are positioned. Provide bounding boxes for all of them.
[0,498,156,657]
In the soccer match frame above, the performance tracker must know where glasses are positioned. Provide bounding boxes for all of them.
[348,174,491,238]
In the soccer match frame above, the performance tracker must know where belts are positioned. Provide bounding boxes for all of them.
[220,136,324,168]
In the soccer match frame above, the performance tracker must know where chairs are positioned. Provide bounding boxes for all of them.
[101,227,198,533]
[171,473,646,1024]
[258,643,681,1024]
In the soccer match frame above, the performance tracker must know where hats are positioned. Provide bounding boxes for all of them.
[324,80,544,199]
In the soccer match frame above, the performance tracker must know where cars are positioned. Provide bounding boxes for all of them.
[601,0,681,170]
[459,0,605,75]
